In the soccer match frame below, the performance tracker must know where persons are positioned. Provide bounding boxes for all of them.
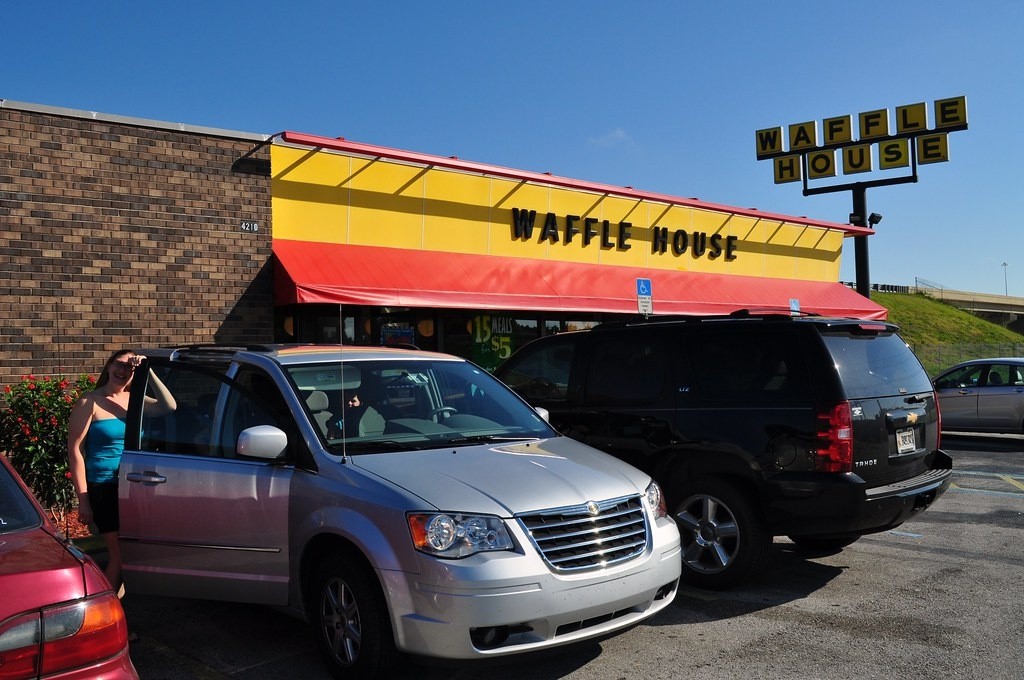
[332,386,366,437]
[66,349,177,646]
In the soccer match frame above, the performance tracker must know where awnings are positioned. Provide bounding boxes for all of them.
[267,240,888,329]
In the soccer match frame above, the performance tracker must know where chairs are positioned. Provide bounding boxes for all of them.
[174,393,249,435]
[988,370,1002,385]
[299,389,339,440]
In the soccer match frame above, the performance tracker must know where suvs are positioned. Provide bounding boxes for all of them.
[113,345,681,676]
[493,314,955,591]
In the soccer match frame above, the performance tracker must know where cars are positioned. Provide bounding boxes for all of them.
[0,454,142,680]
[931,357,1024,436]
